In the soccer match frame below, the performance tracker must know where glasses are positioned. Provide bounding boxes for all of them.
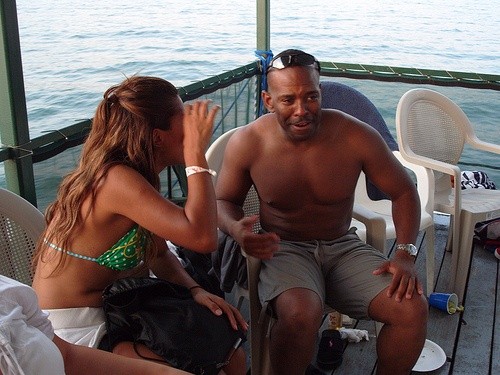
[268,54,321,71]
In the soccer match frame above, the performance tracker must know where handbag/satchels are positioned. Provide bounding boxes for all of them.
[101,278,246,375]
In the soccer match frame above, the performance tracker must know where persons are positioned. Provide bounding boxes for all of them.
[216,49,429,375]
[33,76,249,375]
[0,274,193,375]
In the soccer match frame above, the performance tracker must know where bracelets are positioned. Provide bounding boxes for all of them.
[185,166,217,177]
[189,286,201,290]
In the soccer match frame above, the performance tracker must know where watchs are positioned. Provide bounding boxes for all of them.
[397,244,417,256]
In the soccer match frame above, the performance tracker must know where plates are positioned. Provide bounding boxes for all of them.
[411,338,447,372]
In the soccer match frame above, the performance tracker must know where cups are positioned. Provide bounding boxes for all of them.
[429,292,459,314]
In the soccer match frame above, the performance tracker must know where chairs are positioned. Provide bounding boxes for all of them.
[321,80,434,301]
[205,118,387,375]
[395,86,500,304]
[0,181,50,375]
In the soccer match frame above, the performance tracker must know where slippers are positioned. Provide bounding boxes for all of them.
[316,329,343,370]
